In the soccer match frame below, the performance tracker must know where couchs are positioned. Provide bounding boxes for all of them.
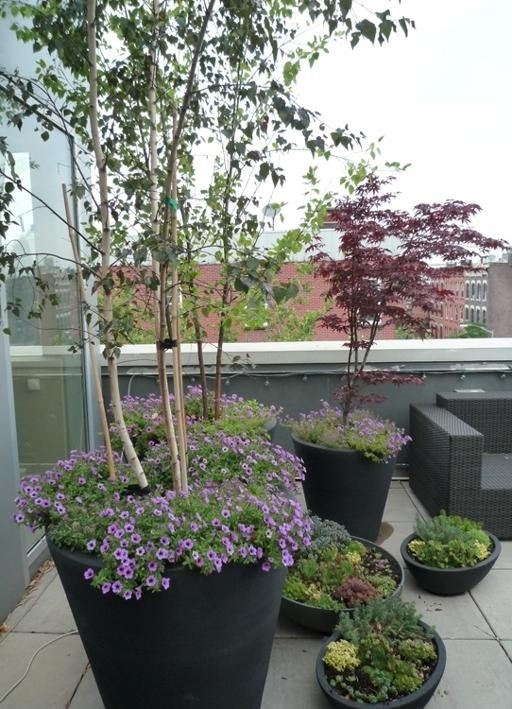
[406,391,509,544]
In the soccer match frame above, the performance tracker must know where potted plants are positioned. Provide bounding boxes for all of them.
[316,591,448,706]
[400,509,501,597]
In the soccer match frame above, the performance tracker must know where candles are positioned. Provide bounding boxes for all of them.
[4,4,417,706]
[288,190,509,544]
